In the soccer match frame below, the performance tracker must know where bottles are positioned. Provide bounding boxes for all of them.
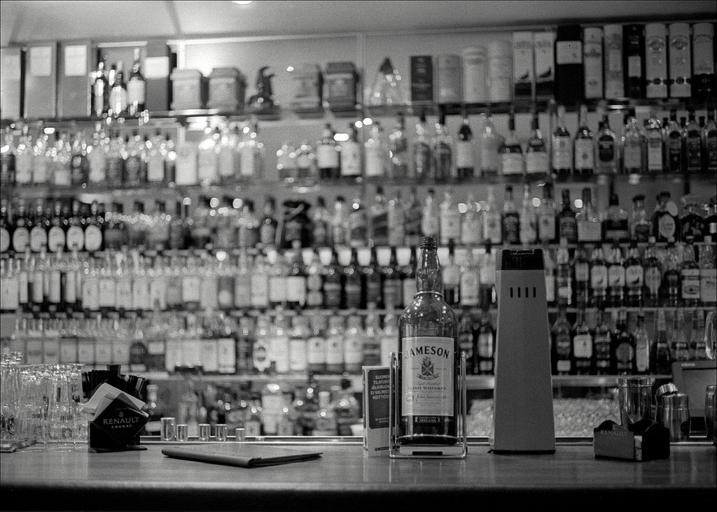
[144,375,364,437]
[1,22,716,375]
[397,238,458,447]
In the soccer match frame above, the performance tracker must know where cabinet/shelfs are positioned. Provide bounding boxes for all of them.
[0,94,716,442]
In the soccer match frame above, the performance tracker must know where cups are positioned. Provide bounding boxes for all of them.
[0,353,92,449]
[466,397,622,436]
